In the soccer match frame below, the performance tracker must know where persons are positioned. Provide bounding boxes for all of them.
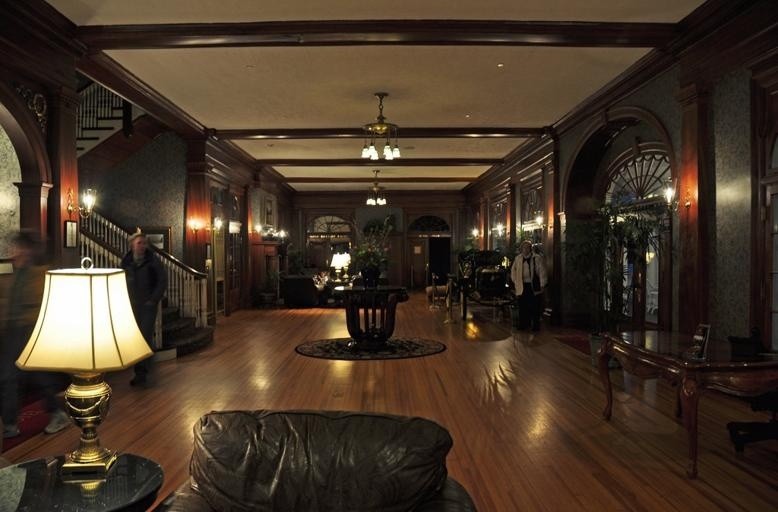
[1,228,71,439]
[118,233,168,385]
[511,239,548,336]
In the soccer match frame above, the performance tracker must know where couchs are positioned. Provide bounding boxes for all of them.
[149,408,480,511]
[279,266,330,306]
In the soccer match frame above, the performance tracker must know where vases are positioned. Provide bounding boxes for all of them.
[361,270,381,287]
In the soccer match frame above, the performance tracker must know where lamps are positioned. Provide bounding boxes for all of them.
[329,252,345,284]
[64,187,98,217]
[13,255,157,480]
[358,91,403,161]
[661,176,695,214]
[365,167,388,206]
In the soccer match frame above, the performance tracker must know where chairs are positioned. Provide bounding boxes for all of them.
[726,332,776,453]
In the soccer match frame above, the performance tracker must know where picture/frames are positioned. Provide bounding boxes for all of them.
[205,243,211,259]
[263,195,274,228]
[64,219,79,249]
[127,225,171,256]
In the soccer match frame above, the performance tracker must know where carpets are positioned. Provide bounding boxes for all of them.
[0,379,75,455]
[293,336,448,361]
[553,331,671,380]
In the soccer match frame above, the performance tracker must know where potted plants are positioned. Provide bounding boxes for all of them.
[254,265,285,306]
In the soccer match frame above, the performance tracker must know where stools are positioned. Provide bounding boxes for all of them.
[423,284,456,306]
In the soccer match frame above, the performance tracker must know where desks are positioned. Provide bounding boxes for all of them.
[333,282,411,353]
[1,451,166,511]
[594,327,777,482]
